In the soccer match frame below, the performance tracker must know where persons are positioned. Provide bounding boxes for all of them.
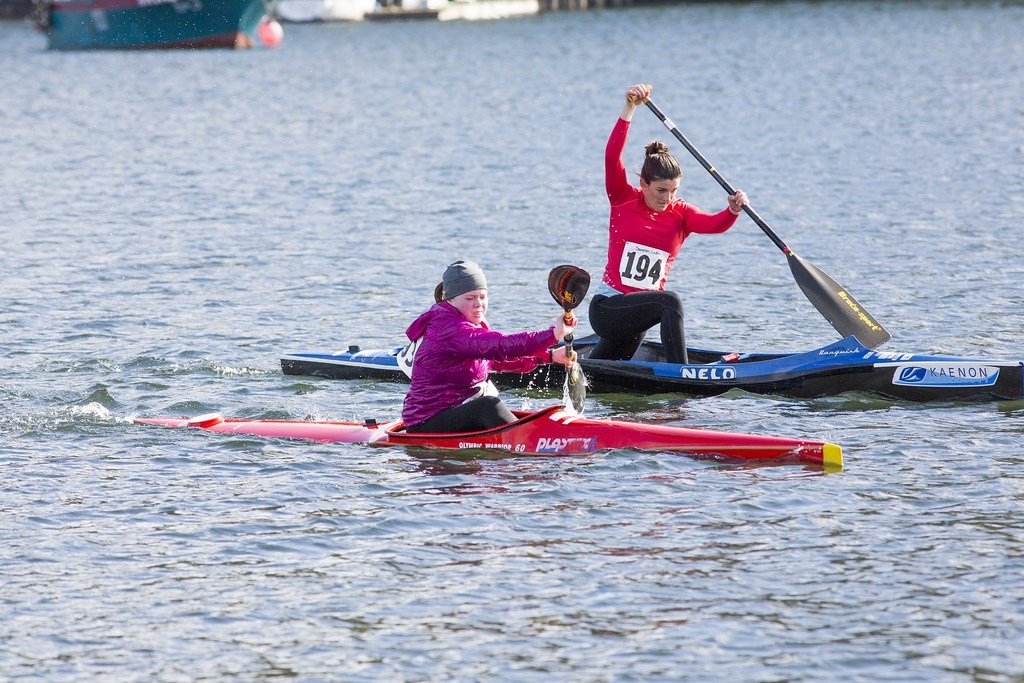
[401,261,577,433]
[589,85,750,363]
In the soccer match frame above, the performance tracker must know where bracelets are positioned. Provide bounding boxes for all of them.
[729,206,740,215]
[550,350,552,362]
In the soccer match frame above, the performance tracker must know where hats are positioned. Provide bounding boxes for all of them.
[442,260,488,301]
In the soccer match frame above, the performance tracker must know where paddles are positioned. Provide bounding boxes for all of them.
[627,83,892,350]
[546,263,591,411]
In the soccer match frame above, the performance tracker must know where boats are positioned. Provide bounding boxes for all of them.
[279,334,1024,397]
[33,0,274,50]
[126,402,845,468]
